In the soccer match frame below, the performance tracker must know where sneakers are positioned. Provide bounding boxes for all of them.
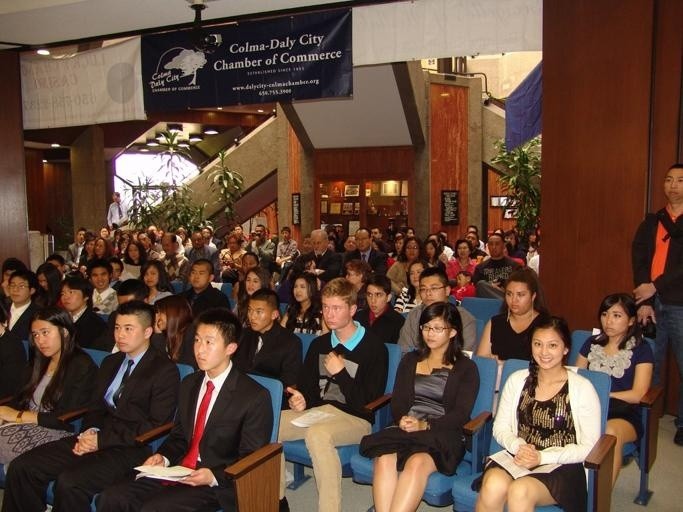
[672,426,682,445]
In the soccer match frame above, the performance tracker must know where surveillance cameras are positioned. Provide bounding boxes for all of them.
[205,34,222,46]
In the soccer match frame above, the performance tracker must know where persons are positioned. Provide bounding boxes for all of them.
[372,301,480,511]
[574,293,654,493]
[1,223,547,404]
[95,307,274,512]
[277,277,389,512]
[1,299,180,510]
[474,314,602,512]
[108,193,128,230]
[632,164,681,448]
[1,306,100,488]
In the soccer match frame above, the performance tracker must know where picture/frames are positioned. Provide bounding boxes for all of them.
[490,195,521,220]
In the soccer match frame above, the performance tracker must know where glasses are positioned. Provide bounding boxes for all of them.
[418,324,450,334]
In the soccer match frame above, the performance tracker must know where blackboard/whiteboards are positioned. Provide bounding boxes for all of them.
[440,190,460,226]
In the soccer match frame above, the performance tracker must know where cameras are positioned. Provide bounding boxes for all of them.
[642,316,656,339]
[252,232,260,236]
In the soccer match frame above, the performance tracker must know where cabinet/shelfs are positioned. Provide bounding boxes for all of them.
[313,176,409,240]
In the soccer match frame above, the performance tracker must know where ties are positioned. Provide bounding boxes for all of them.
[161,381,214,486]
[116,203,122,220]
[112,360,133,407]
[253,336,263,356]
[361,254,366,261]
